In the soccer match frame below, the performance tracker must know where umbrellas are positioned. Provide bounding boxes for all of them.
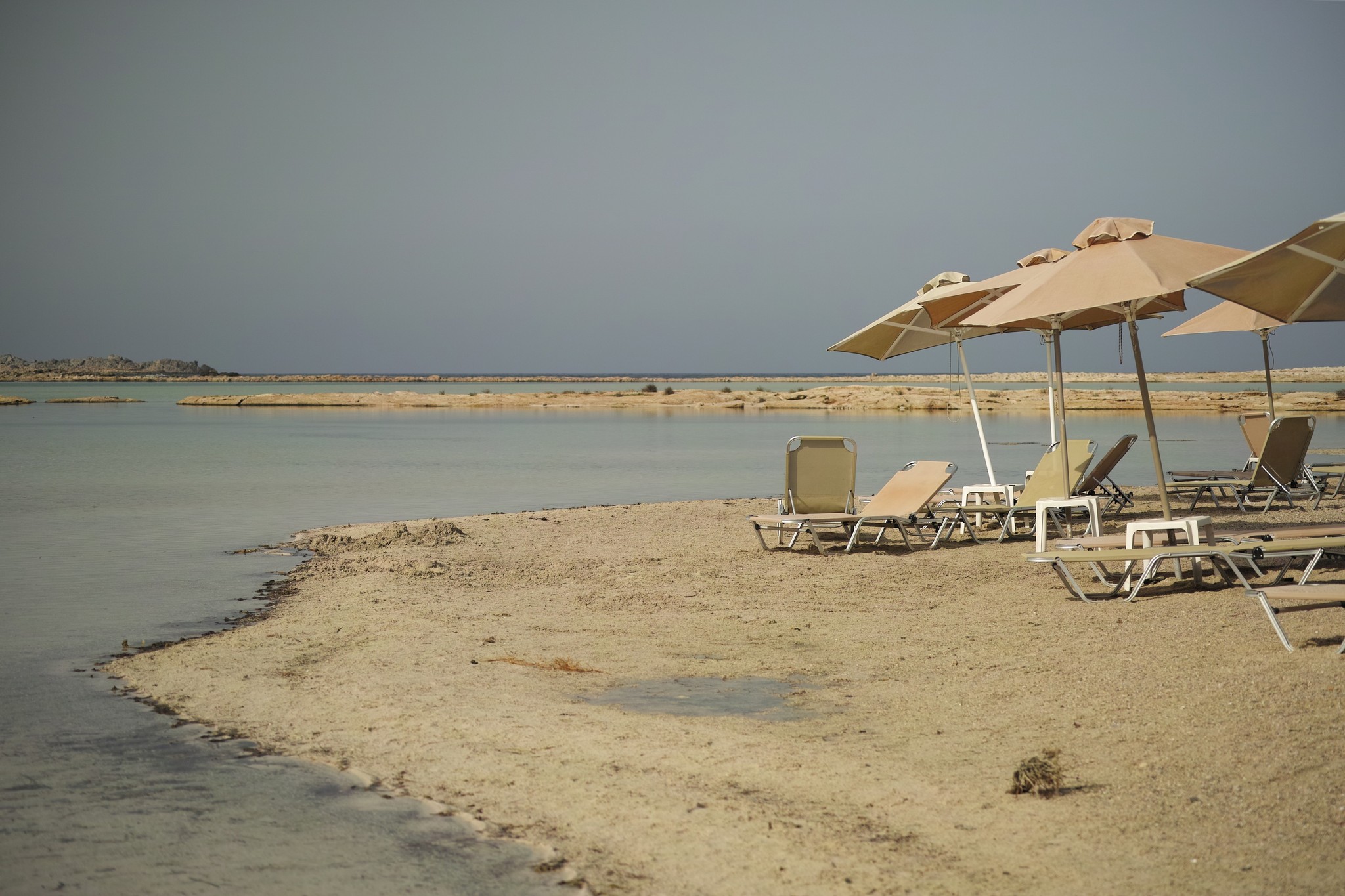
[918,248,1187,539]
[827,270,1030,527]
[1187,211,1345,323]
[958,216,1256,590]
[1161,299,1298,423]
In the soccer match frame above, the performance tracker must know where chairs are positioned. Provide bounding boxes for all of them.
[745,412,1345,655]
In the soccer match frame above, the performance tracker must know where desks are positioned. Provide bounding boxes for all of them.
[1035,495,1104,553]
[960,484,1029,534]
[1124,516,1221,591]
